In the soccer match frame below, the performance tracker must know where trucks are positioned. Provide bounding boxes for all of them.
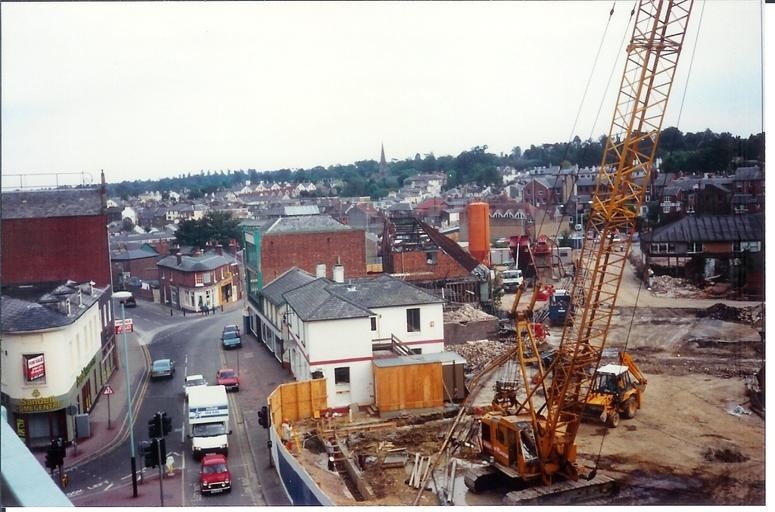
[186,385,233,460]
[502,235,540,293]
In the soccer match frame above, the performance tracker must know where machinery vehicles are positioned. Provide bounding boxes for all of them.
[413,217,535,342]
[463,0,696,506]
[554,351,648,428]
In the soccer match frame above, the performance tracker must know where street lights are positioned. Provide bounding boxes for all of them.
[111,290,137,500]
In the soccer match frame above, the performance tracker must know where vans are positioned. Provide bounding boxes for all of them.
[547,289,574,324]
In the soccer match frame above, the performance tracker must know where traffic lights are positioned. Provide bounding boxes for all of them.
[148,410,174,439]
[138,438,167,469]
[257,406,272,428]
[45,438,68,469]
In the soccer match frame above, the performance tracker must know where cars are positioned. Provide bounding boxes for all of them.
[125,292,136,308]
[216,368,240,392]
[223,324,241,337]
[182,374,209,398]
[220,332,242,350]
[150,359,176,379]
[199,453,233,495]
